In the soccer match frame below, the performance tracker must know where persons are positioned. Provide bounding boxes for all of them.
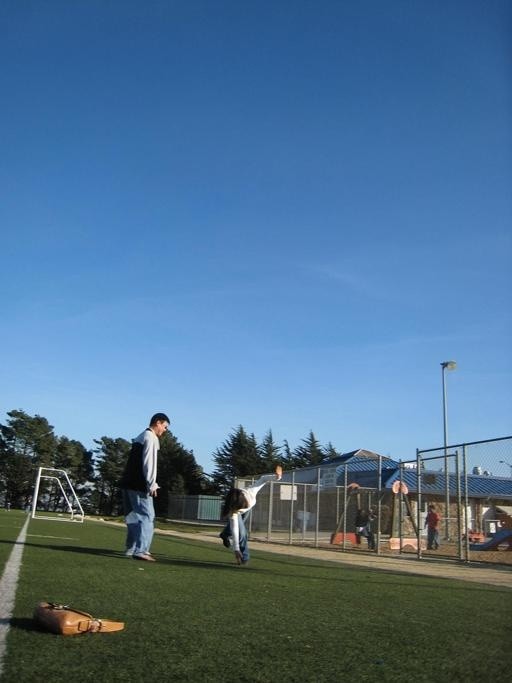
[355,508,375,551]
[219,465,283,566]
[424,505,441,551]
[121,413,170,559]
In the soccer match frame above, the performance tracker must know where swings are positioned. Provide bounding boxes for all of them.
[353,487,386,535]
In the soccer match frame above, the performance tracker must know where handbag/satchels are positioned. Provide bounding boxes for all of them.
[34,601,94,635]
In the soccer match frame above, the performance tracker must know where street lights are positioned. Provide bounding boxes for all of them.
[500,460,512,468]
[440,361,456,539]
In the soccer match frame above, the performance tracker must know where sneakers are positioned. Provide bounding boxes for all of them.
[133,553,156,561]
[220,533,231,547]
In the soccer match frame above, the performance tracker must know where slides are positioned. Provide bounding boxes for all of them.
[469,528,511,551]
